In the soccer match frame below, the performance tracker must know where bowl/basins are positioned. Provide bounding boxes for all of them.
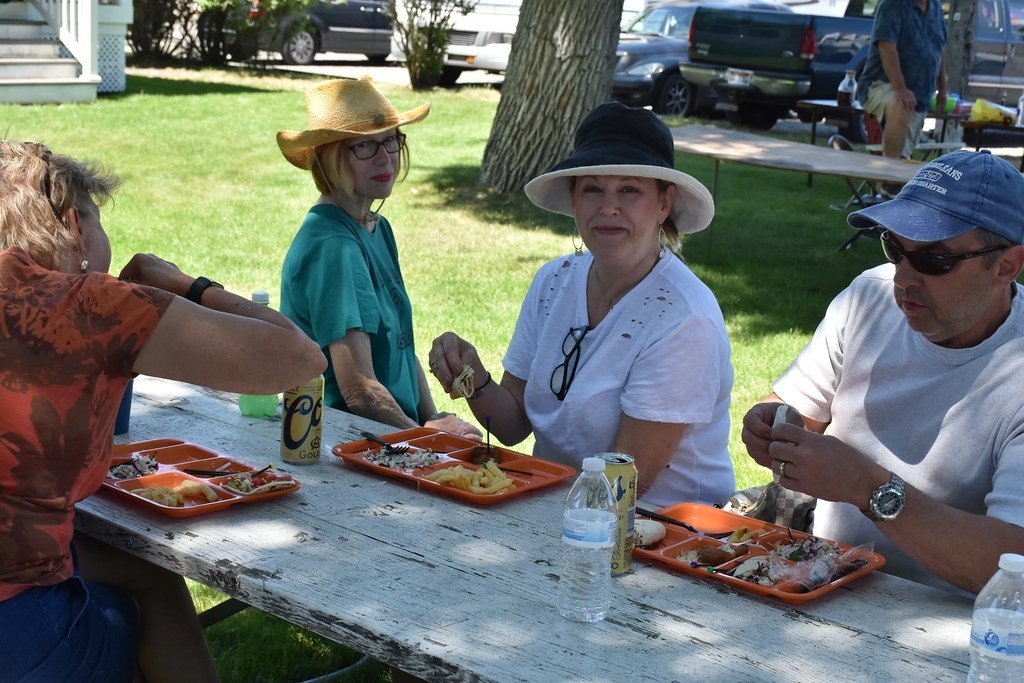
[929,96,957,116]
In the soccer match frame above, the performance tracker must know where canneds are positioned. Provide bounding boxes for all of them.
[279,373,325,466]
[588,453,638,576]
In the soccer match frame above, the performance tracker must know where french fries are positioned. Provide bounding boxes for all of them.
[728,525,768,543]
[424,461,517,494]
[130,479,219,506]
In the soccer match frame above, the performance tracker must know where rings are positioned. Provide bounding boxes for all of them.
[430,360,435,367]
[778,462,786,477]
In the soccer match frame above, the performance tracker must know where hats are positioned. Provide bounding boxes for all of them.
[523,101,715,233]
[276,74,431,170]
[847,150,1024,253]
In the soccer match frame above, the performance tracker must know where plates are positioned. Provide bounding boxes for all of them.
[631,502,886,605]
[101,437,302,518]
[331,427,576,505]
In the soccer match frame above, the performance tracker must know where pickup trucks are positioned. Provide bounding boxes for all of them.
[675,0,1024,131]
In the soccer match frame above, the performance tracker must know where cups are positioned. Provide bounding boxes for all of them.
[114,378,134,435]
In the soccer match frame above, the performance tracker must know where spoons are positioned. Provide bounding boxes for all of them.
[635,506,735,539]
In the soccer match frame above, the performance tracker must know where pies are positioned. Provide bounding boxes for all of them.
[449,365,475,400]
[633,519,666,549]
[772,404,788,428]
[221,480,295,496]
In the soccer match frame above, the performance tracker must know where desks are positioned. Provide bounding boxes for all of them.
[959,116,1024,172]
[797,99,971,188]
[669,124,931,262]
[75,373,979,683]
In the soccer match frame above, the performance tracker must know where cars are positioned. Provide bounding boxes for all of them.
[390,0,647,85]
[196,0,396,65]
[612,0,801,131]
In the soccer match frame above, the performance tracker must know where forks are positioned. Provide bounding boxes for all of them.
[361,431,410,455]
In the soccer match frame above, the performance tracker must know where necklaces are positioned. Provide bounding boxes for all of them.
[594,248,664,306]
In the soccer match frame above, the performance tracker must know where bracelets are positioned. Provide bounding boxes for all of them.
[474,371,491,390]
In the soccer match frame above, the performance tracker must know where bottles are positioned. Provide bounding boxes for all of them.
[556,457,619,622]
[837,70,858,107]
[965,552,1024,683]
[1014,91,1024,128]
[237,290,279,418]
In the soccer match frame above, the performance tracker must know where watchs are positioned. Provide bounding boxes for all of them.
[185,276,224,306]
[860,471,906,523]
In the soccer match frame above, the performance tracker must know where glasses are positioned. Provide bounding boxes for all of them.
[23,141,68,230]
[549,325,588,402]
[880,228,1008,276]
[331,132,406,160]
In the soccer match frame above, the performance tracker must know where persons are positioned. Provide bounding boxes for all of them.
[857,0,948,198]
[429,101,736,511]
[742,147,1024,602]
[276,75,484,442]
[0,139,329,683]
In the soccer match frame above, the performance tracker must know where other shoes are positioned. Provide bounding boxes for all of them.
[878,182,904,199]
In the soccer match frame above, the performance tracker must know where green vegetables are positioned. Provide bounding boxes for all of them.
[785,545,806,562]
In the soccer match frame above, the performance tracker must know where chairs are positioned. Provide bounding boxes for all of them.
[828,135,965,223]
